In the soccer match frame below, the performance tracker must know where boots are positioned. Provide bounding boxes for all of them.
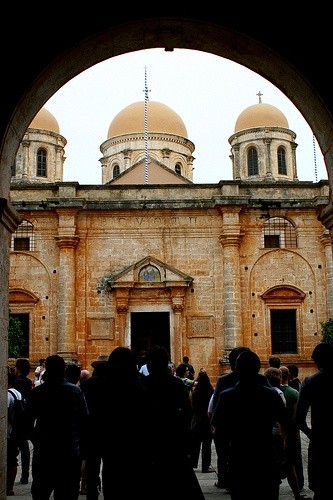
[7,467,18,496]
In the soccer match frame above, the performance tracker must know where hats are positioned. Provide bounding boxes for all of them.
[90,355,109,368]
[80,369,90,378]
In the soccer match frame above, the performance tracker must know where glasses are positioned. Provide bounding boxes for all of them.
[186,369,189,372]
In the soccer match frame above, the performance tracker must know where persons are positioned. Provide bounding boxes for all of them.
[7,346,312,500]
[294,342,333,500]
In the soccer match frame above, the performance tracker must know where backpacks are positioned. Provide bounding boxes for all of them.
[8,388,33,440]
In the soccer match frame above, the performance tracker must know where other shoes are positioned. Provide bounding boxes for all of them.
[295,497,312,500]
[20,477,29,484]
[215,482,220,487]
[203,467,215,473]
[80,491,86,495]
